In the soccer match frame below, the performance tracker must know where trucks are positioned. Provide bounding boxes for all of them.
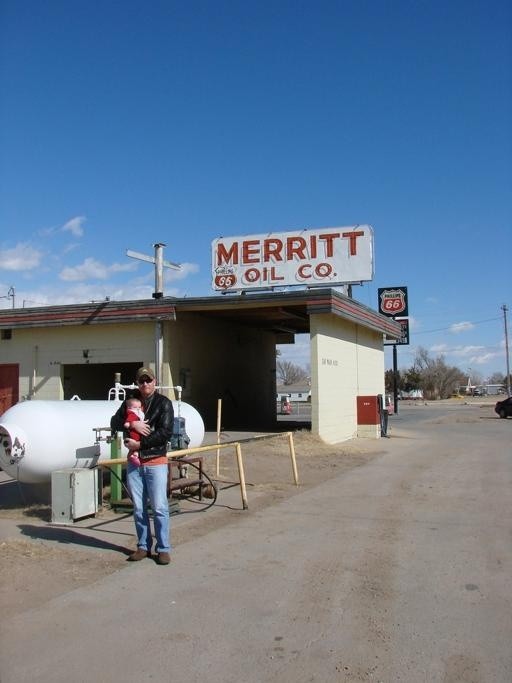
[473,387,483,397]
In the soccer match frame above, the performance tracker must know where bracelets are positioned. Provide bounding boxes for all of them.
[128,420,134,428]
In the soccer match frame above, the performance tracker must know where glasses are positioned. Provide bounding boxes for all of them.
[138,379,152,384]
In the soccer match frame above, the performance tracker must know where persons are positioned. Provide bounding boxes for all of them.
[122,397,147,468]
[110,365,176,566]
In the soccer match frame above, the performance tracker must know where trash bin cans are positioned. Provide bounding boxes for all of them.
[382,409,388,436]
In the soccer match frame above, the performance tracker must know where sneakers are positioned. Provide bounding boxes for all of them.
[130,549,151,561]
[158,552,171,564]
[127,452,141,466]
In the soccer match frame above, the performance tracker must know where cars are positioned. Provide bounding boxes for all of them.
[495,396,512,419]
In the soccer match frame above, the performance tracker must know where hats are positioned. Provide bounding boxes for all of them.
[136,367,154,381]
